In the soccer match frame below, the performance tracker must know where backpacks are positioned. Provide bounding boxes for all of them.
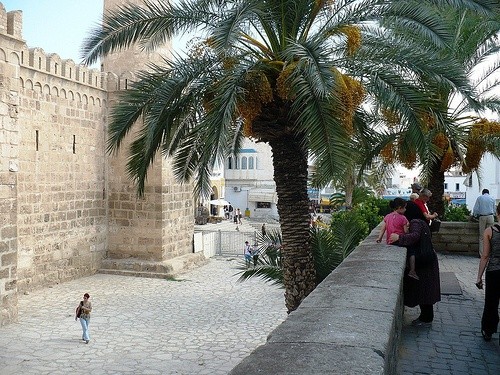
[76,301,84,318]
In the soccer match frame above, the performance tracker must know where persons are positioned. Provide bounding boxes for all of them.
[476,201,500,341]
[389,208,442,327]
[376,197,421,282]
[234,207,239,225]
[472,188,497,259]
[262,223,267,239]
[251,242,261,268]
[238,207,242,225]
[243,240,252,268]
[213,201,233,220]
[75,293,92,343]
[413,186,439,260]
[407,191,429,214]
[406,183,431,220]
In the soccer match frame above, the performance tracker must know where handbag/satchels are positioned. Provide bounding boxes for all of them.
[431,220,441,232]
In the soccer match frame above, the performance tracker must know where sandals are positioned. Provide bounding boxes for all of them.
[408,271,420,280]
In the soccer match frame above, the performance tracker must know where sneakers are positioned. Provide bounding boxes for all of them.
[411,317,421,326]
[411,320,432,328]
[481,329,492,340]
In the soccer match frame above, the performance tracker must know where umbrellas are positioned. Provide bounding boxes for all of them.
[208,198,231,222]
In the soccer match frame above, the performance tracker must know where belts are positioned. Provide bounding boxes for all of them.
[480,213,493,216]
